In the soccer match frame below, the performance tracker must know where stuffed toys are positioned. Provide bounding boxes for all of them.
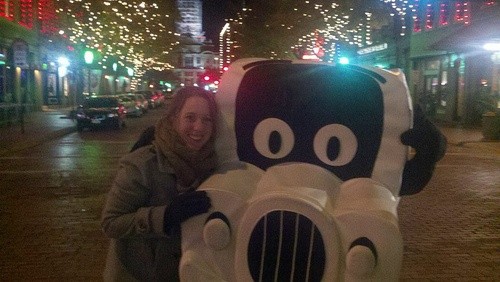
[180,58,442,281]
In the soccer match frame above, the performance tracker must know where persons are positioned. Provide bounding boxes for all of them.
[100,87,216,282]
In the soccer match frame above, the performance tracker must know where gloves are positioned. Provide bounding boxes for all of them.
[163,189,212,233]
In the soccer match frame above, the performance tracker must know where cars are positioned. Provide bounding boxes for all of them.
[117,94,149,117]
[154,91,166,104]
[136,90,161,109]
[76,96,128,134]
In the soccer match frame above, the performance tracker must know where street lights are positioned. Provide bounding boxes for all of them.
[83,50,94,98]
